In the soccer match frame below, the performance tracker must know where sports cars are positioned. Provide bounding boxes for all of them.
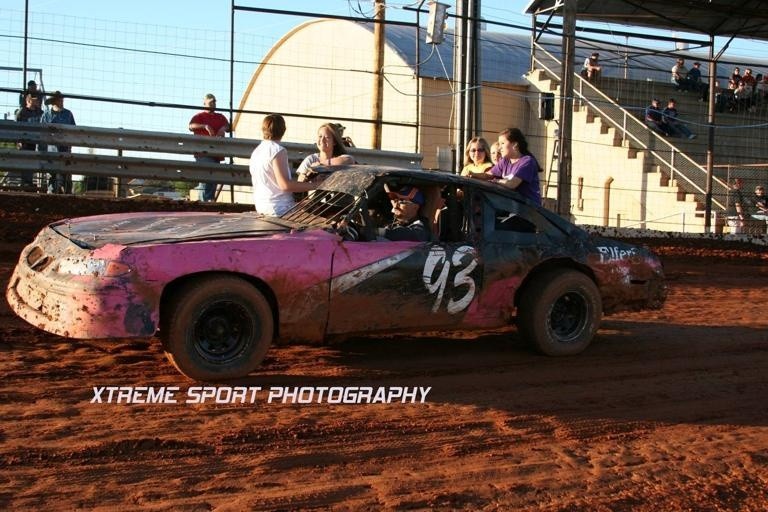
[6,163,669,384]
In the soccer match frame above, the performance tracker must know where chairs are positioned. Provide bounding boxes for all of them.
[423,186,459,244]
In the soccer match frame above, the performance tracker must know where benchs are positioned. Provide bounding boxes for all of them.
[577,72,768,238]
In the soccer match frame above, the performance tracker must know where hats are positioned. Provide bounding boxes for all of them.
[387,187,424,206]
[44,91,63,106]
[204,93,216,107]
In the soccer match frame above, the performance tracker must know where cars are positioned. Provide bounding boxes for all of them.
[3,177,43,193]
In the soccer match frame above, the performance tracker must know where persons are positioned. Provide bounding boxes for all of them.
[644,52,768,240]
[16,93,44,186]
[581,52,602,83]
[189,94,230,204]
[249,113,544,247]
[20,80,42,107]
[40,91,77,193]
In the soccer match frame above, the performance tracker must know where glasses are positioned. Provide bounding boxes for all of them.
[470,147,485,154]
